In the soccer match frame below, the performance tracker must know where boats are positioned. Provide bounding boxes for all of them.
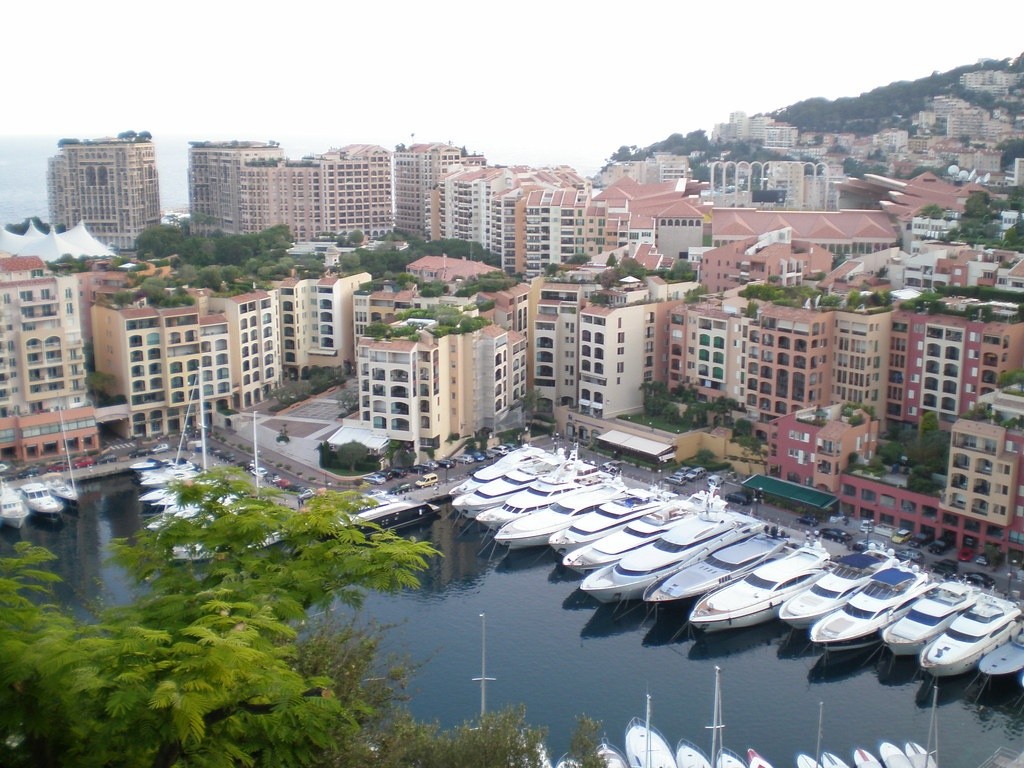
[440,424,1023,768]
[0,367,264,562]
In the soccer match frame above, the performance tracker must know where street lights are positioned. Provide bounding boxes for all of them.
[866,519,874,551]
[1007,571,1011,594]
[1010,560,1016,573]
[445,463,451,485]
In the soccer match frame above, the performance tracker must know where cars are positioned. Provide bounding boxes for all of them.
[263,472,282,485]
[409,464,430,475]
[470,451,486,462]
[456,454,474,464]
[150,444,169,453]
[73,455,94,469]
[274,479,292,490]
[420,461,439,470]
[92,453,118,465]
[501,442,518,451]
[128,448,151,459]
[438,459,457,468]
[46,462,69,472]
[297,489,314,501]
[359,466,408,486]
[283,483,305,492]
[388,482,412,495]
[482,448,496,459]
[250,467,268,479]
[18,464,40,480]
[360,489,387,498]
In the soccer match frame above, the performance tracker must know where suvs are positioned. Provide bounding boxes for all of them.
[415,473,438,489]
[487,444,509,455]
